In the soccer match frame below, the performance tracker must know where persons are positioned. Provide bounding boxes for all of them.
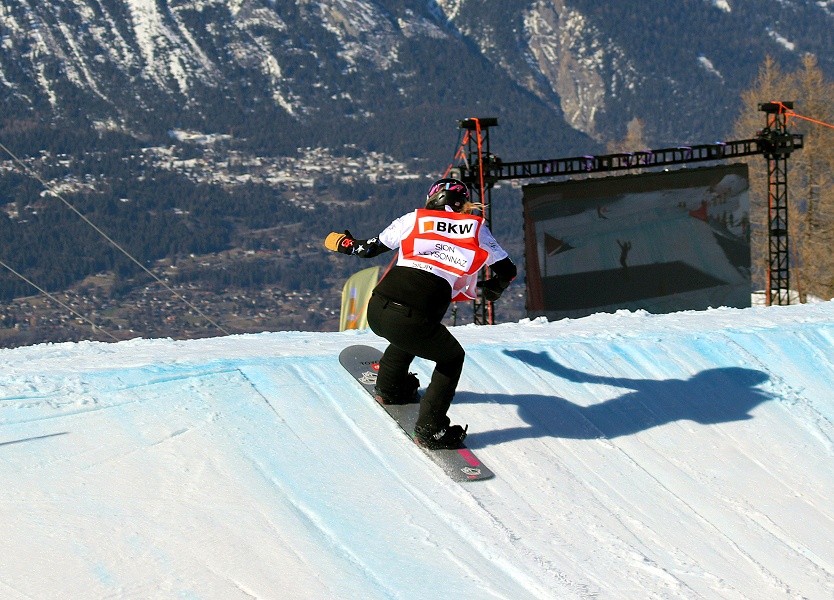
[322,176,519,449]
[617,238,632,269]
[595,204,603,219]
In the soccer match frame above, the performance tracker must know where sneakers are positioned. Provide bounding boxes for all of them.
[414,424,468,449]
[375,372,420,405]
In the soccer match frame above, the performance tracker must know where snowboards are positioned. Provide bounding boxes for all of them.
[338,343,496,483]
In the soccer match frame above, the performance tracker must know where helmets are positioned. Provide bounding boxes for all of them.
[425,178,470,212]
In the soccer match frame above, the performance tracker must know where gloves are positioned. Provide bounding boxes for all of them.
[324,230,355,254]
[477,275,510,301]
